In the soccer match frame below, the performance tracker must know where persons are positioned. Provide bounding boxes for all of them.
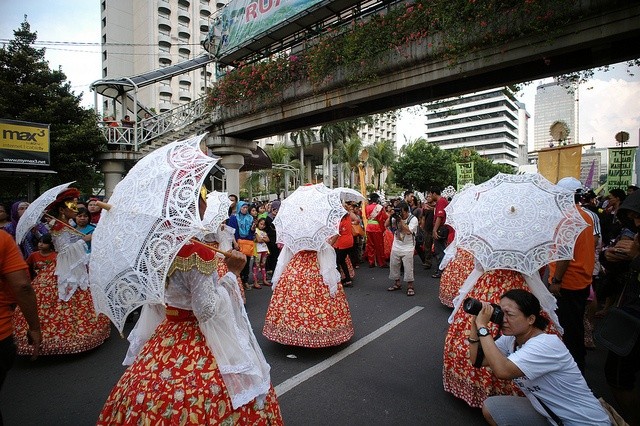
[255,202,264,216]
[383,203,394,262]
[385,205,419,295]
[412,197,422,215]
[579,205,599,332]
[253,217,273,288]
[265,202,273,218]
[74,200,86,212]
[443,266,563,409]
[346,204,360,270]
[140,113,153,146]
[400,192,414,214]
[228,199,252,293]
[85,194,101,225]
[264,200,282,278]
[328,214,354,287]
[218,226,246,304]
[94,192,284,426]
[422,192,435,270]
[385,208,396,219]
[104,116,119,151]
[12,189,111,355]
[391,198,399,206]
[0,204,10,228]
[0,229,42,396]
[556,176,595,372]
[365,193,388,268]
[8,201,49,277]
[439,248,474,308]
[228,195,238,217]
[609,189,623,213]
[248,205,258,288]
[25,233,55,276]
[121,115,135,152]
[69,207,96,273]
[599,189,640,416]
[627,186,636,197]
[262,251,355,348]
[354,202,363,253]
[462,289,611,426]
[430,187,448,279]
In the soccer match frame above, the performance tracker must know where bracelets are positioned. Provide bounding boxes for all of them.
[467,336,479,343]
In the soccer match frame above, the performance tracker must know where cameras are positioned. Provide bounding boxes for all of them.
[389,207,401,223]
[463,297,504,325]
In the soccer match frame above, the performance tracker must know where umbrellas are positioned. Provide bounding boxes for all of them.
[332,187,368,202]
[88,132,223,338]
[275,182,350,251]
[15,180,86,243]
[444,172,591,278]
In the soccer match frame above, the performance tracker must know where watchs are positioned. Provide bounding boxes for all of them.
[477,327,490,337]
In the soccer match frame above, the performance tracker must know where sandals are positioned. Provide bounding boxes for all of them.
[254,284,263,288]
[432,273,441,277]
[407,286,415,296]
[388,284,402,291]
[264,283,272,286]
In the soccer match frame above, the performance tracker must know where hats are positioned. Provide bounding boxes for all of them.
[556,177,584,195]
[45,187,80,210]
